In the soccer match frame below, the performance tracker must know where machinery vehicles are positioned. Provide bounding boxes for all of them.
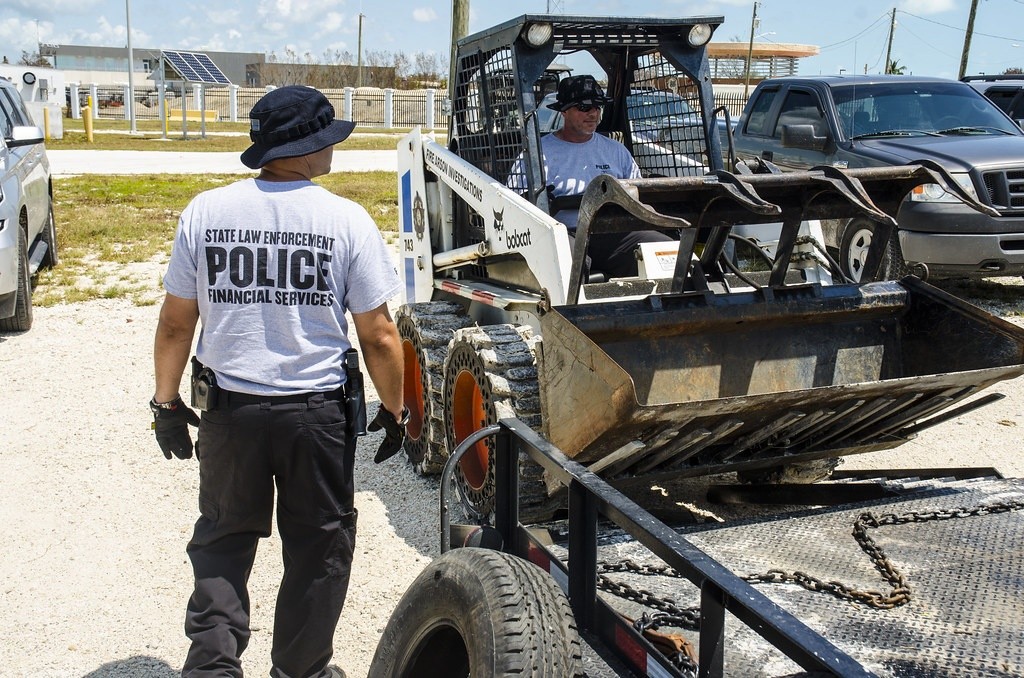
[393,14,1024,530]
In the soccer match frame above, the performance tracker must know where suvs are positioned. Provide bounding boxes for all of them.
[367,418,1024,678]
[0,77,57,330]
[956,75,1024,144]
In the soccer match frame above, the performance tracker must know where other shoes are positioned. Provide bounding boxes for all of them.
[269,664,347,678]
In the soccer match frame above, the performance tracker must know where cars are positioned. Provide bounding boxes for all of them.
[528,91,698,148]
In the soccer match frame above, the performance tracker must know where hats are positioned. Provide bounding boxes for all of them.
[239,85,357,169]
[546,75,614,114]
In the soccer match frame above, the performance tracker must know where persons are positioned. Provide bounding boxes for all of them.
[505,76,645,234]
[150,87,413,677]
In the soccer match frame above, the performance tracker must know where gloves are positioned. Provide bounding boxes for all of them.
[149,395,201,460]
[368,402,411,464]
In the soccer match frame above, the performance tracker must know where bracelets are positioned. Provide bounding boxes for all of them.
[152,395,181,409]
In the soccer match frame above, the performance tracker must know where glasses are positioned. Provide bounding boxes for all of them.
[570,103,604,112]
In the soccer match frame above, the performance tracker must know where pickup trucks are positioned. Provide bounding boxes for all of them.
[659,73,1024,296]
